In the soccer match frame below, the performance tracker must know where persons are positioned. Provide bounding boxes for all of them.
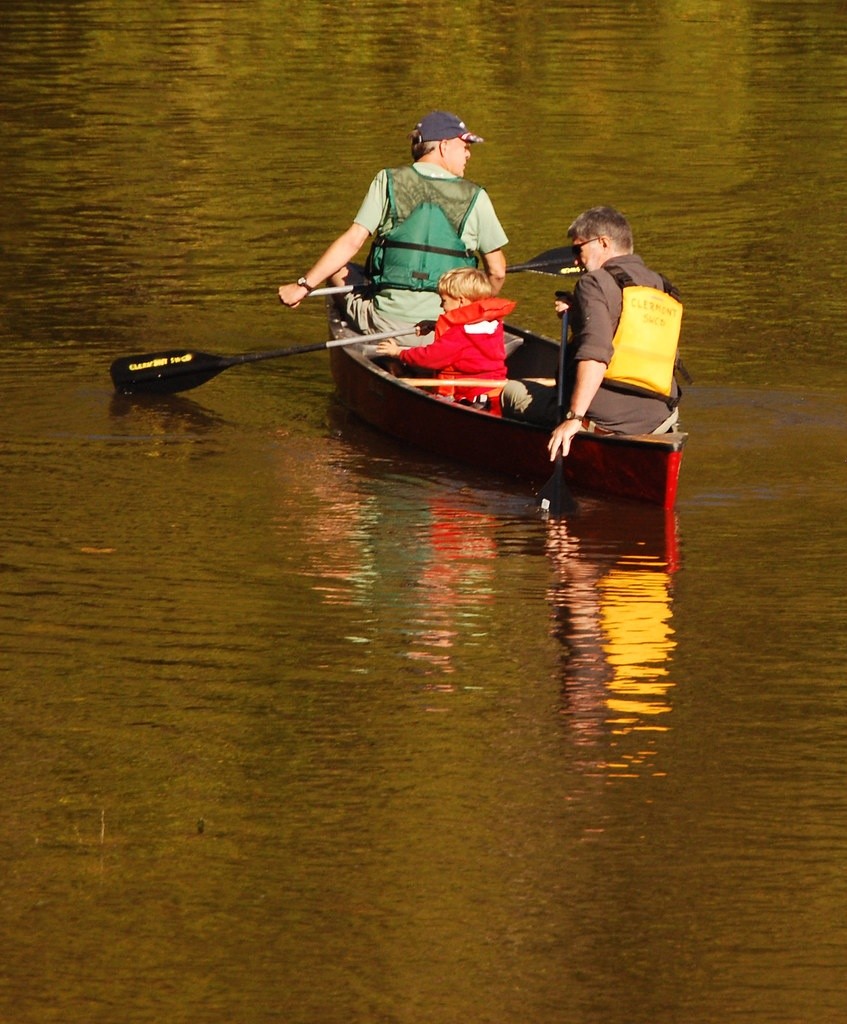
[376,266,516,417]
[278,111,508,348]
[500,205,682,460]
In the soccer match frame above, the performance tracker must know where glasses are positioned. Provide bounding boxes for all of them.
[571,238,600,258]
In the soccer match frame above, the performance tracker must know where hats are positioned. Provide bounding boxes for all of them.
[413,113,484,144]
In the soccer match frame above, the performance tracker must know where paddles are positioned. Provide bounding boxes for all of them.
[304,244,588,298]
[108,328,417,395]
[536,290,579,518]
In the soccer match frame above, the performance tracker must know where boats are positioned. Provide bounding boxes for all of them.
[326,281,690,509]
[323,403,681,577]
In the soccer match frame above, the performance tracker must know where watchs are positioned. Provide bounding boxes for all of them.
[297,276,314,292]
[566,410,583,422]
